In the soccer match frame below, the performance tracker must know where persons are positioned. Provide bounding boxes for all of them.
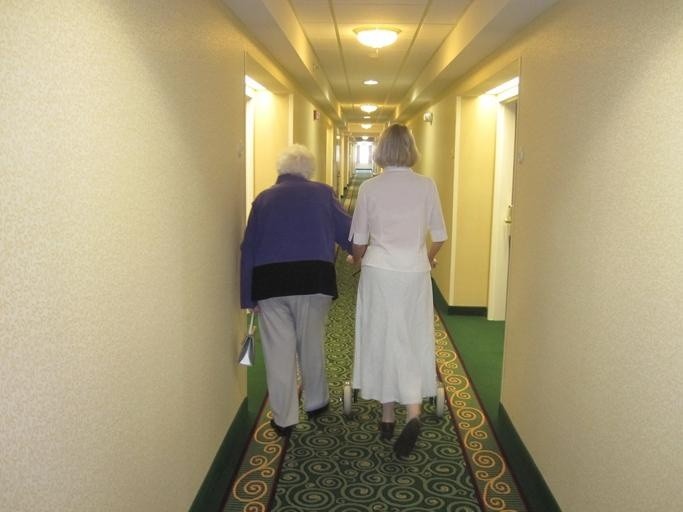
[238,143,353,439]
[346,122,447,458]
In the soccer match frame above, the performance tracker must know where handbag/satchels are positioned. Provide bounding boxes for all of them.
[239,324,257,368]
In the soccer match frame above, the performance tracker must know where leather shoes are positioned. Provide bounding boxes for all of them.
[394,418,419,456]
[271,419,292,437]
[307,402,329,418]
[380,421,396,439]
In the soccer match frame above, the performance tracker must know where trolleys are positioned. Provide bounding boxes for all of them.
[336,256,446,422]
